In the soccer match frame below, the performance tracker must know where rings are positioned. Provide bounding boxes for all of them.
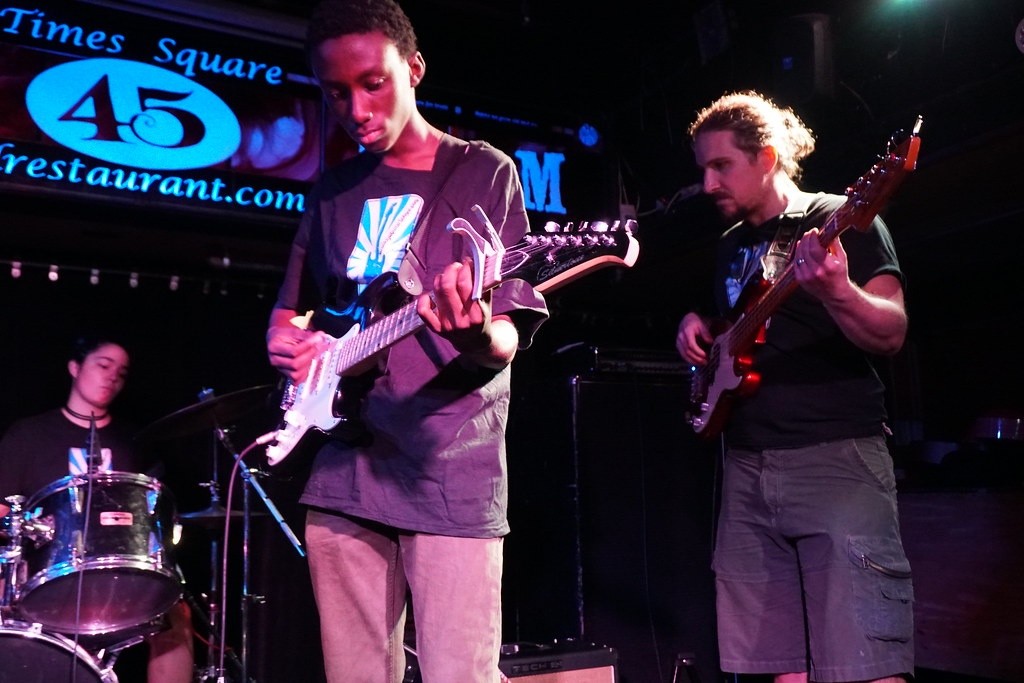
[797,257,805,266]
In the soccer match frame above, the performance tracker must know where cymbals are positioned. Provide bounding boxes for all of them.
[136,382,273,446]
[176,502,266,530]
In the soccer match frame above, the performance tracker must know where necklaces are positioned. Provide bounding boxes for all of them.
[64,404,108,421]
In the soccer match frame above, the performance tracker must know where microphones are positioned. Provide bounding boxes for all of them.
[85,432,102,468]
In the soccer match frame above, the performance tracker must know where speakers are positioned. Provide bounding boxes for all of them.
[498,646,628,683]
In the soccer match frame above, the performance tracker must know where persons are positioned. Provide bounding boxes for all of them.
[677,90,915,683]
[0,333,195,683]
[267,0,548,683]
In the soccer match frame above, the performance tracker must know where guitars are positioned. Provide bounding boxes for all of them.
[681,111,927,443]
[247,218,642,468]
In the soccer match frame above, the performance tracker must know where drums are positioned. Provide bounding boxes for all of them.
[6,473,185,638]
[0,624,113,683]
